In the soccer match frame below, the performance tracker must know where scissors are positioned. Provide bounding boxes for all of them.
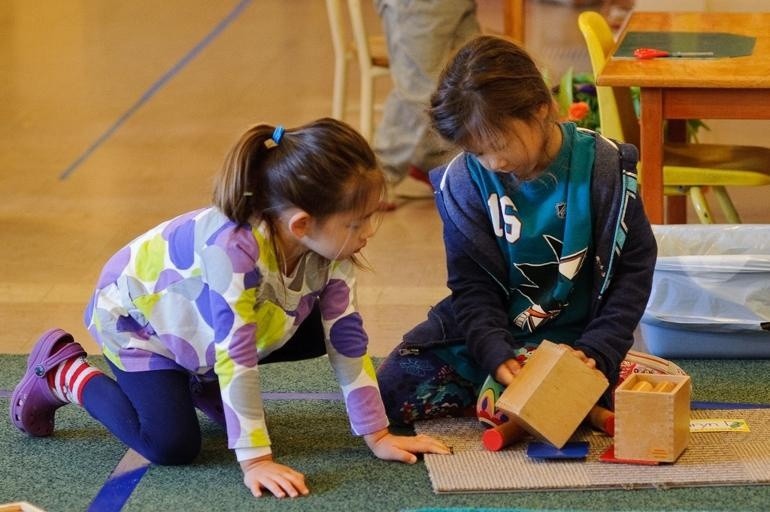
[633,47,715,59]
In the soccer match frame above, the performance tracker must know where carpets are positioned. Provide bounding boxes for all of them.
[0,354,770,512]
[412,407,770,495]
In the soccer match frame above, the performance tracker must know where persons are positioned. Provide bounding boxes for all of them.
[10,117,453,500]
[368,2,485,214]
[374,37,692,438]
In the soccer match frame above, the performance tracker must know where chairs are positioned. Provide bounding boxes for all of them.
[325,0,391,145]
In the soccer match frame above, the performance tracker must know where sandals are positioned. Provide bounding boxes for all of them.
[11,329,85,437]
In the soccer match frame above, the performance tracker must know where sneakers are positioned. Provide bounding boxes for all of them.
[621,350,692,398]
